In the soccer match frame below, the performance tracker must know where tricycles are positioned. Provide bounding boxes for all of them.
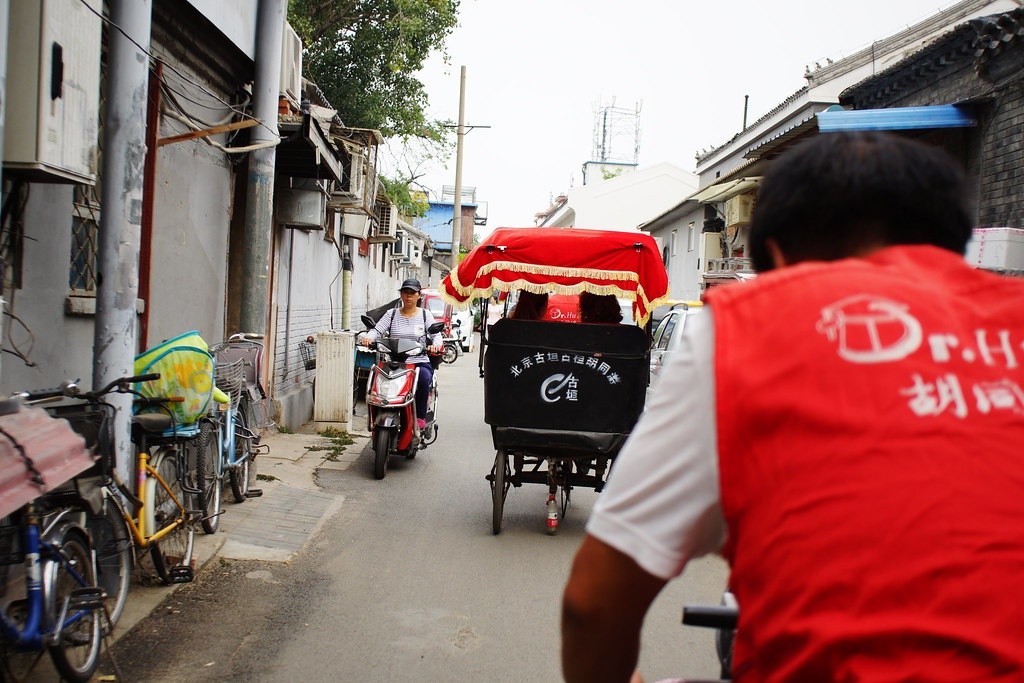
[479,226,652,535]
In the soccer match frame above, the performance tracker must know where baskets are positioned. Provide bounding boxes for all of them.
[214,357,244,411]
[299,342,316,370]
[44,403,116,481]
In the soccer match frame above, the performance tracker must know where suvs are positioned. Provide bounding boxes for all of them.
[644,302,703,408]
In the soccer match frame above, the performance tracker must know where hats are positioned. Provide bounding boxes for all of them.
[401,279,420,291]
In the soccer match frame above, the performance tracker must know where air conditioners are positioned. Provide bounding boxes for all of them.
[400,240,415,263]
[331,145,363,199]
[409,250,422,269]
[725,194,756,228]
[392,230,409,256]
[376,204,398,239]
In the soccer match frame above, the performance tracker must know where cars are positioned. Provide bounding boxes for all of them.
[421,288,474,352]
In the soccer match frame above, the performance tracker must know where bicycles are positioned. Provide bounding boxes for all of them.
[0,332,278,683]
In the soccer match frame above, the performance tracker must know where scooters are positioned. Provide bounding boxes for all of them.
[441,330,461,364]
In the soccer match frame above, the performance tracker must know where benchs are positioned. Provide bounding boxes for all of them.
[484,318,651,459]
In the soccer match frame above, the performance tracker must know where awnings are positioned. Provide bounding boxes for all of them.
[686,175,768,220]
[741,106,979,165]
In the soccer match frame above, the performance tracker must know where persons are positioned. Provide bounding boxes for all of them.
[359,277,446,430]
[559,126,1023,683]
[541,291,582,324]
[509,288,548,321]
[487,295,503,338]
[575,290,625,327]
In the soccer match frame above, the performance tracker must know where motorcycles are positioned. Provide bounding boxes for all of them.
[355,314,445,480]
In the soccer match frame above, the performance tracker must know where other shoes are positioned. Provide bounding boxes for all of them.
[417,418,426,429]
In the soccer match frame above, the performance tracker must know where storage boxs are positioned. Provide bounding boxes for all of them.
[964,227,1024,272]
